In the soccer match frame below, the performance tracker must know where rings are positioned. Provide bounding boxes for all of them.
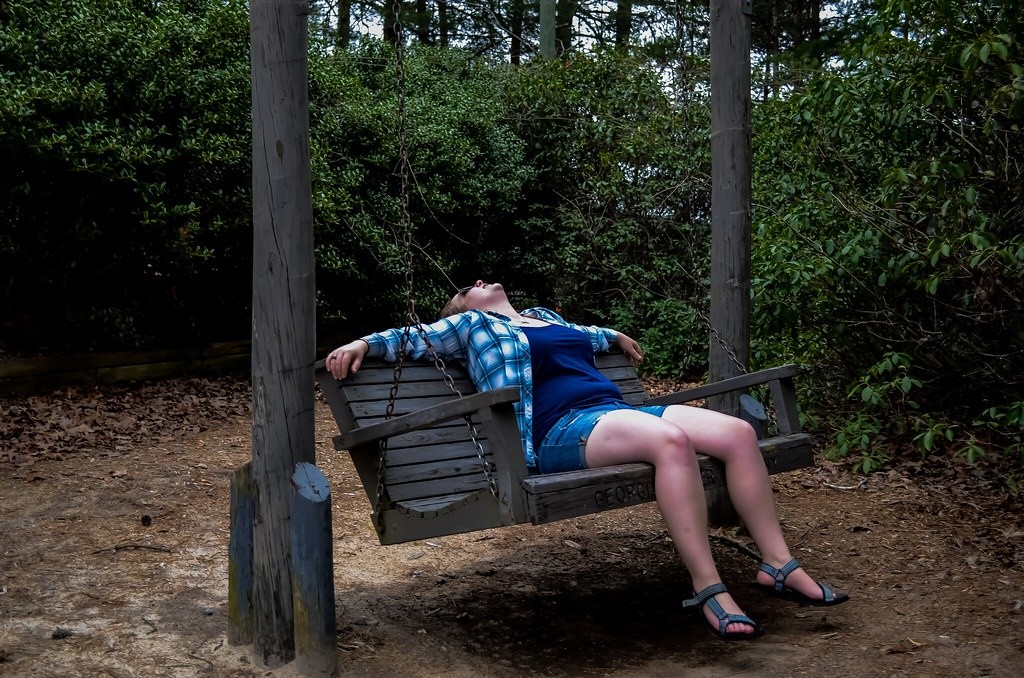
[331,355,337,360]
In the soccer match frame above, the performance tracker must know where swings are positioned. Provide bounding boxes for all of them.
[310,0,820,550]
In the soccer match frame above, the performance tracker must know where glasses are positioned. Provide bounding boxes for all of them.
[456,285,474,314]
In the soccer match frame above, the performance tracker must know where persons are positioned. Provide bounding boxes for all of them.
[325,278,851,638]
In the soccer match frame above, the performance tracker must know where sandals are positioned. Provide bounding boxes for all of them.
[755,558,850,606]
[683,580,760,641]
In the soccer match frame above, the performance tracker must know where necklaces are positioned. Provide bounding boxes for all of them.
[518,318,532,324]
